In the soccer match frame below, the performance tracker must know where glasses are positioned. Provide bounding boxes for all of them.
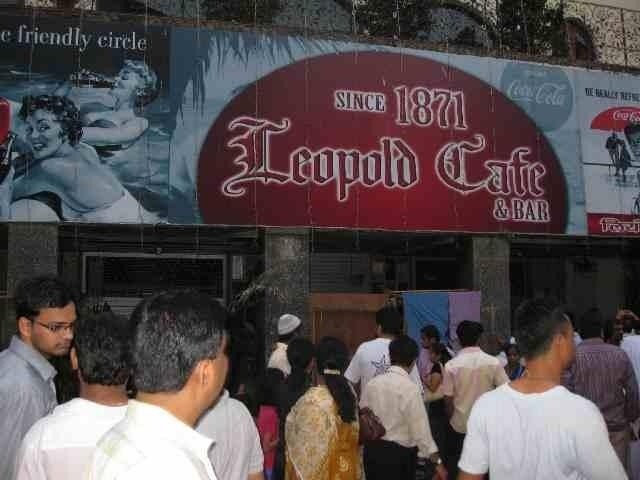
[31,320,75,332]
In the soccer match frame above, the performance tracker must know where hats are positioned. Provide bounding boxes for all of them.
[278,314,301,335]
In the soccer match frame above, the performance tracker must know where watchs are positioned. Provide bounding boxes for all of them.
[431,458,445,470]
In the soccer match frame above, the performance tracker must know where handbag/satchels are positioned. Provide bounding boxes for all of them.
[359,406,386,446]
[422,362,445,403]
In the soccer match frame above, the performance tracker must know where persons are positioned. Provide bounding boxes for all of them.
[504,343,528,382]
[3,59,159,147]
[423,319,512,480]
[613,143,631,183]
[416,325,457,384]
[358,335,449,480]
[0,271,77,480]
[494,332,512,368]
[605,132,624,166]
[455,294,629,480]
[343,305,426,408]
[560,301,640,480]
[78,288,230,478]
[284,336,368,480]
[0,165,15,221]
[255,367,288,479]
[192,387,266,480]
[623,120,640,186]
[272,337,315,479]
[11,312,137,480]
[420,341,453,480]
[610,309,640,480]
[266,313,305,378]
[12,94,170,224]
